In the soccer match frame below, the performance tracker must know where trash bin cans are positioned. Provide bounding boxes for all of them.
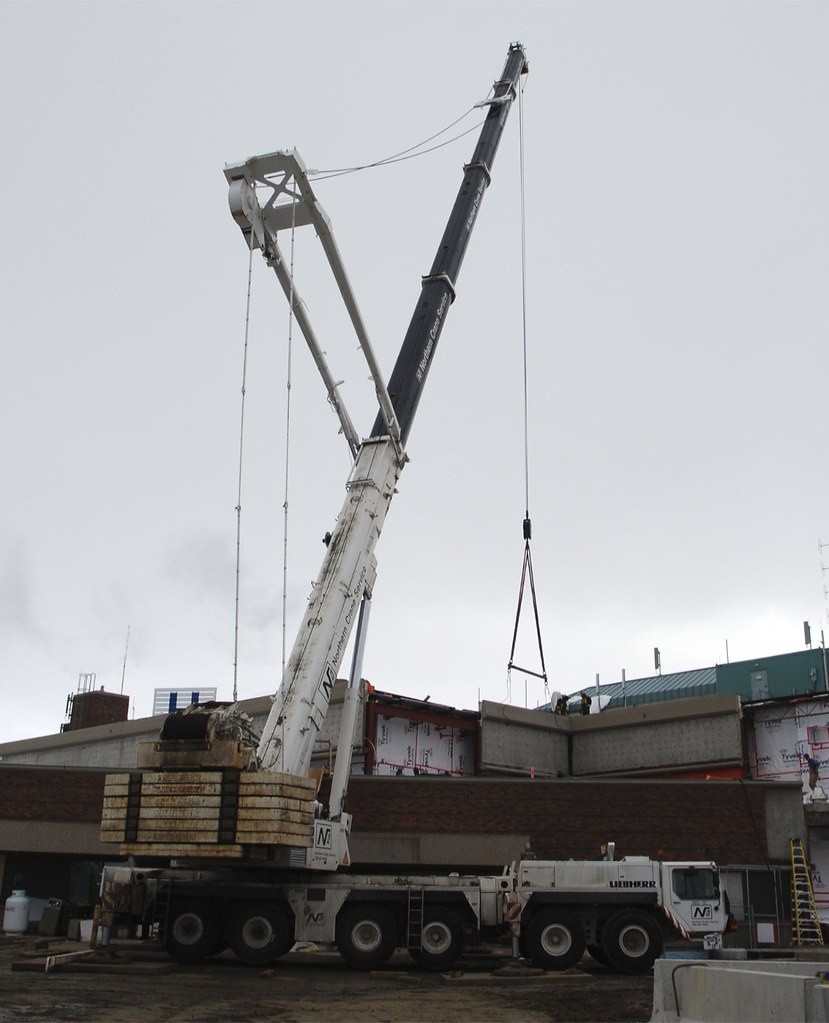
[38,897,72,938]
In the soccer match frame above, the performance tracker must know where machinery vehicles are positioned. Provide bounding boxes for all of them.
[81,44,735,973]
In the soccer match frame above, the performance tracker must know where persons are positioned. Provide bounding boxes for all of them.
[555,695,571,715]
[579,693,591,715]
[804,753,819,790]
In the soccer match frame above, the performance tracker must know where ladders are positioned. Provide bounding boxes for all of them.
[788,836,824,946]
